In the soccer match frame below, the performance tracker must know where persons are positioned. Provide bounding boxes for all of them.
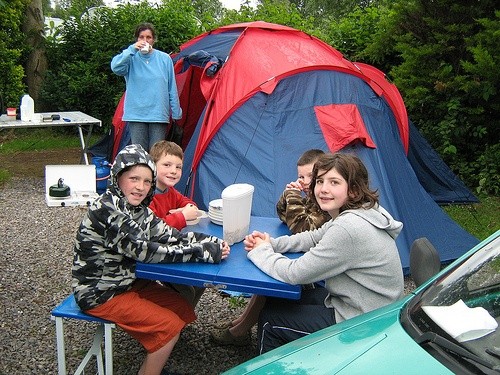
[243,152,405,358]
[207,148,332,345]
[111,24,185,153]
[72,140,230,375]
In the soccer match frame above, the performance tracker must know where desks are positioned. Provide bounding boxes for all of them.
[0,112,101,168]
[135,214,301,301]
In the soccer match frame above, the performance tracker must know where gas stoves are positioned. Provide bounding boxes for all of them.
[45,192,100,207]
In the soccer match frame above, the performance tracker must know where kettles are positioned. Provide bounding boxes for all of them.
[48,178,71,197]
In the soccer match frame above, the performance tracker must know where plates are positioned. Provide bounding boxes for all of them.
[208,198,223,226]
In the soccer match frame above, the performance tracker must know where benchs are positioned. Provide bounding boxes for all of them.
[51,290,115,375]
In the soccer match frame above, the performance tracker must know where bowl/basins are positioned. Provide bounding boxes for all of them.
[169,207,207,226]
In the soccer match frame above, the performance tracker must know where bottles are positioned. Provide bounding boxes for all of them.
[20,94,35,122]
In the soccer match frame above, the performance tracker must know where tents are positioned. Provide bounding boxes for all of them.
[113,19,485,273]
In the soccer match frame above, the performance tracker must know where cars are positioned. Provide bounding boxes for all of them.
[219,229,500,375]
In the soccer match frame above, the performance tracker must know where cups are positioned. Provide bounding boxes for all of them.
[7,108,16,121]
[221,183,255,246]
[140,43,152,55]
